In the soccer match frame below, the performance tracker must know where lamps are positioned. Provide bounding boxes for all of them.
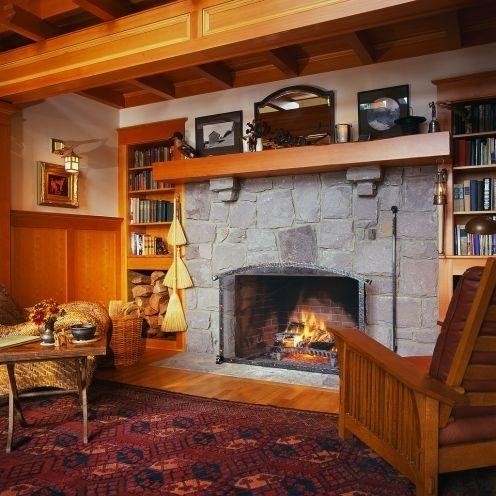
[47,131,108,174]
[432,166,448,205]
[464,211,496,236]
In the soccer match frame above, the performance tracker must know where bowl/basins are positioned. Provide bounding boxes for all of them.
[70,323,96,339]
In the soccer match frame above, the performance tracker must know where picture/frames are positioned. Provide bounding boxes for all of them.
[35,159,78,209]
[194,108,244,158]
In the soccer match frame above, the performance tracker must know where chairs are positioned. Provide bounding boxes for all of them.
[0,280,112,404]
[324,254,495,495]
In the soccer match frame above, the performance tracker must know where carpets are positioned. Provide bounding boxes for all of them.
[1,378,495,496]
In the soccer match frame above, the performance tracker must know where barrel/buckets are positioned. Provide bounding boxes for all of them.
[335,123,351,142]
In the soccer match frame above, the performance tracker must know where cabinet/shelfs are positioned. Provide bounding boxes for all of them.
[430,66,495,340]
[114,116,188,351]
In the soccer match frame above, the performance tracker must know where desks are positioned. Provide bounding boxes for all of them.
[0,334,109,455]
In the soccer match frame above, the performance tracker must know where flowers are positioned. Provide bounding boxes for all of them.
[30,296,67,331]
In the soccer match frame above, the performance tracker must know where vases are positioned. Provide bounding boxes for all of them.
[39,320,57,346]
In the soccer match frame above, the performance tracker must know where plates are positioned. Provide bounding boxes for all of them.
[71,336,102,343]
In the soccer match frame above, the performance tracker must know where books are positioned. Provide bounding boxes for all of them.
[129,146,174,256]
[451,103,496,256]
[0,335,41,350]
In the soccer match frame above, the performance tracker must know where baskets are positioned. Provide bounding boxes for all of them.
[95,317,145,367]
